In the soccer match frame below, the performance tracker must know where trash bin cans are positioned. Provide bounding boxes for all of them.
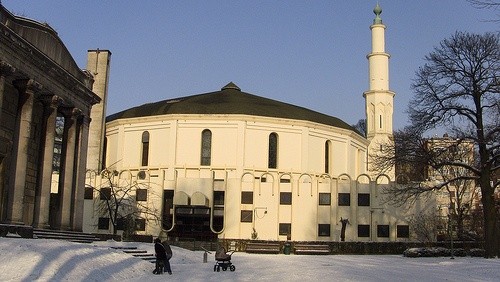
[283,244,292,255]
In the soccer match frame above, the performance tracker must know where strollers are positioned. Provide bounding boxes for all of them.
[213,246,236,272]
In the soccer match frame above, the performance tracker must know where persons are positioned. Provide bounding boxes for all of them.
[279,241,286,255]
[217,251,230,259]
[152,238,172,275]
